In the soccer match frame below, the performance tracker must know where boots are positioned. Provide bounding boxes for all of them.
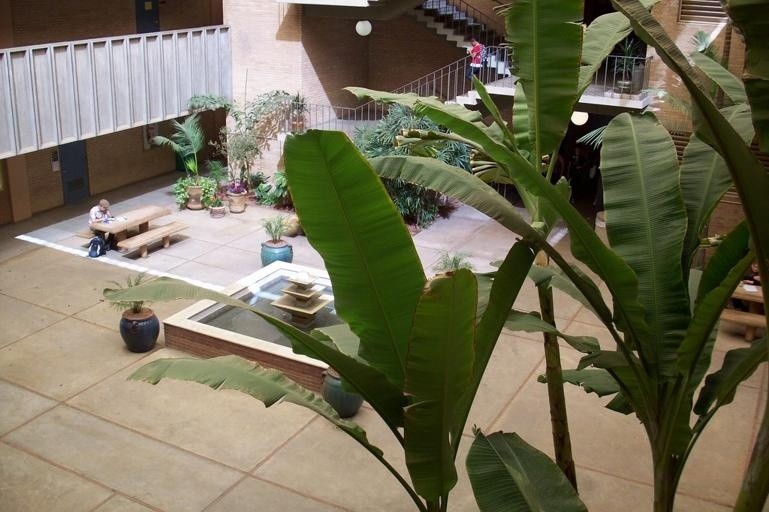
[105,236,112,251]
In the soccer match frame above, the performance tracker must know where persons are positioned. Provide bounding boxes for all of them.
[88,199,114,245]
[732,262,761,308]
[465,35,483,81]
[551,148,602,207]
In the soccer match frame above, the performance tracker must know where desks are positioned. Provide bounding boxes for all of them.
[729,284,764,314]
[89,204,172,240]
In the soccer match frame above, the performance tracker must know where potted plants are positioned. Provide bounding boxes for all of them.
[613,37,642,94]
[260,213,292,267]
[151,89,309,218]
[103,272,160,352]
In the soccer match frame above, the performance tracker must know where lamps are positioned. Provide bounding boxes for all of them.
[354,20,374,37]
[571,110,590,127]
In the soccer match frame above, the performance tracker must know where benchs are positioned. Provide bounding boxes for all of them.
[80,231,96,239]
[719,309,767,341]
[116,222,191,258]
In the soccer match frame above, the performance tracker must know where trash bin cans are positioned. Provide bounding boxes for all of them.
[631,62,644,94]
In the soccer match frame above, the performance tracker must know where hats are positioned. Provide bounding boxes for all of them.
[100,199,110,209]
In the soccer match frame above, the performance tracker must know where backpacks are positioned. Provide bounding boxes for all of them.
[477,43,488,64]
[88,236,104,258]
